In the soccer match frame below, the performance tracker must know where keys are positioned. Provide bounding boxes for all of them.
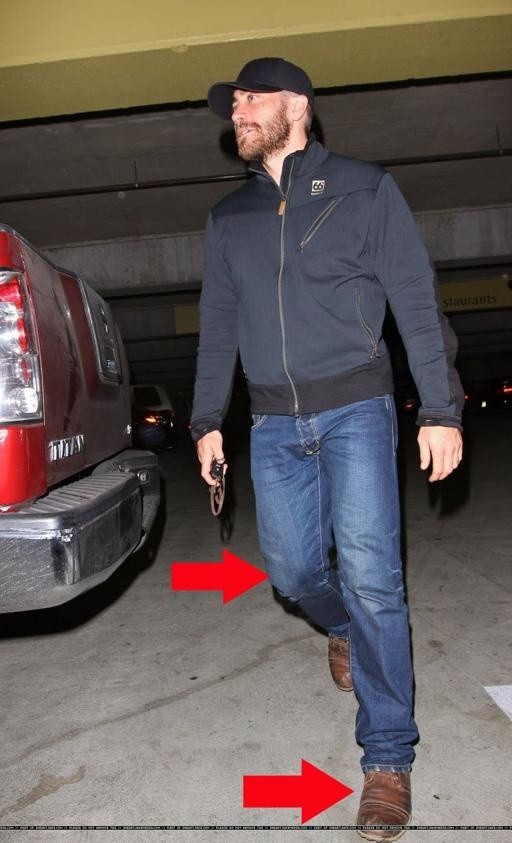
[210,459,224,481]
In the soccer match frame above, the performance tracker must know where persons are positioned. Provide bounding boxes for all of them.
[186,54,472,839]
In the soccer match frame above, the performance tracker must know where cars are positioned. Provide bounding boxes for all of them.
[395,376,512,420]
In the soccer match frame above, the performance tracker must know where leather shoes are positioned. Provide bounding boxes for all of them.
[356,768,413,843]
[327,632,354,691]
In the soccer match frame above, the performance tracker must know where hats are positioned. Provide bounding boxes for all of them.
[207,57,316,120]
